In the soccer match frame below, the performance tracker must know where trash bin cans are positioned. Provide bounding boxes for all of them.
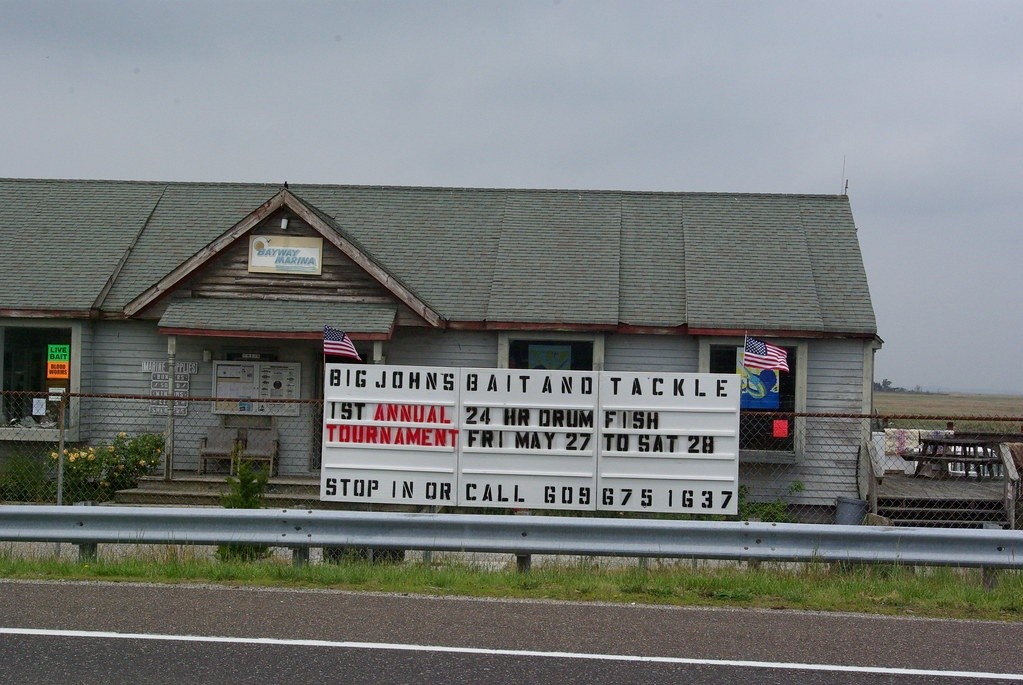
[835,496,868,526]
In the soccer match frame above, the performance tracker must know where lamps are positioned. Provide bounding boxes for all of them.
[380,356,387,365]
[280,214,288,230]
[203,349,212,362]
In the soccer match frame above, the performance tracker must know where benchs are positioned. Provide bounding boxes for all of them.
[901,453,1001,464]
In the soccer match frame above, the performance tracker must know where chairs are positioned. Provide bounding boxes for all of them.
[197,427,238,476]
[235,426,278,478]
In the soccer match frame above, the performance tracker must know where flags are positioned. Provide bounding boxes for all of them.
[324,325,361,360]
[744,337,788,372]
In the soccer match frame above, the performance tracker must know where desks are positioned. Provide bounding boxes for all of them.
[913,439,999,480]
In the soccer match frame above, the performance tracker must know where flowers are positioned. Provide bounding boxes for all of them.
[43,431,167,488]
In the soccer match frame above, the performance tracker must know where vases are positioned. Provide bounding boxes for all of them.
[82,483,115,502]
